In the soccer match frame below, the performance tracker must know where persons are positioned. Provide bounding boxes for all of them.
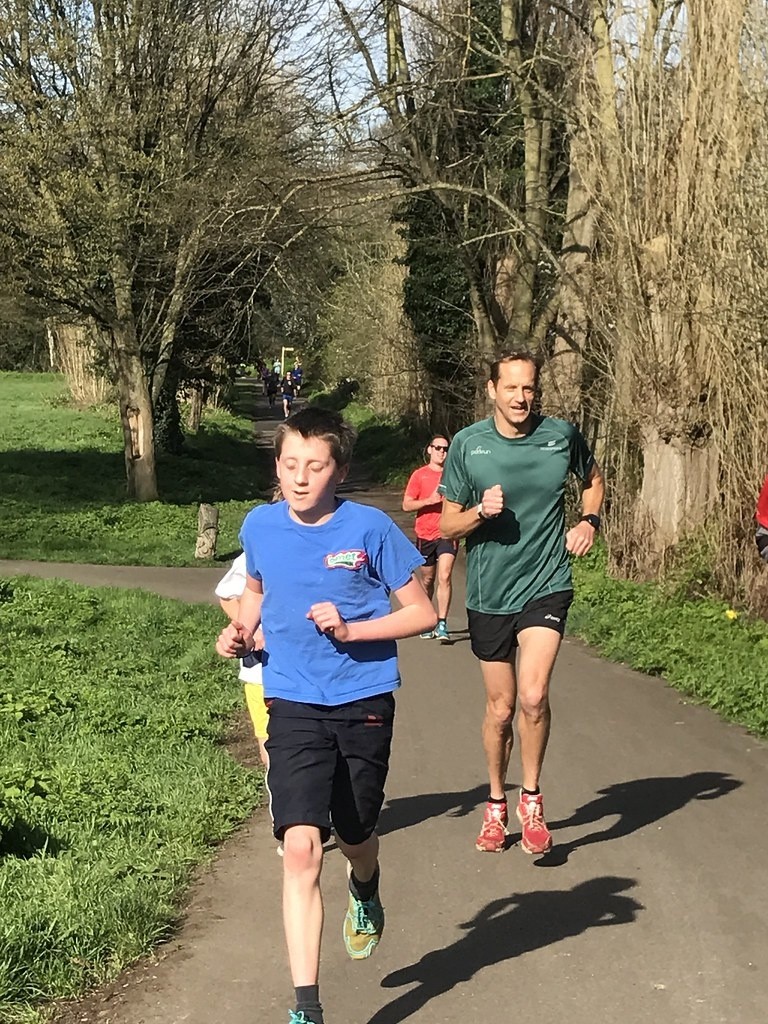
[402,434,459,642]
[439,349,605,855]
[215,405,437,1024]
[258,356,304,420]
[215,552,286,856]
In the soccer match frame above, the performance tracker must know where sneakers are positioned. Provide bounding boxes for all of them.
[475,801,508,853]
[516,788,552,854]
[342,859,384,961]
[288,1009,315,1024]
[434,621,450,641]
[419,632,434,639]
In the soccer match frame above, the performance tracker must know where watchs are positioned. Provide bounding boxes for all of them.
[579,513,601,531]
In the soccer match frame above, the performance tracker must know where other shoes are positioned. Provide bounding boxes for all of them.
[277,840,284,856]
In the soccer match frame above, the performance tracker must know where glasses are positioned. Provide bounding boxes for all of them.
[430,445,448,452]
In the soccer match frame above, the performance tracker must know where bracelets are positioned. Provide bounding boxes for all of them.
[478,503,492,522]
